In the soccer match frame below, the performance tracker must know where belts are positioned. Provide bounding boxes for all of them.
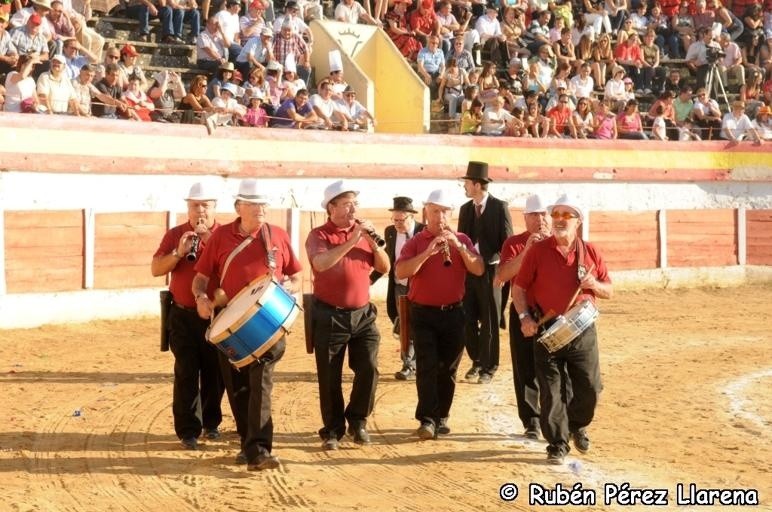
[414,302,463,312]
[320,302,359,313]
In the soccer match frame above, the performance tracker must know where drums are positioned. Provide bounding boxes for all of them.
[536,298,600,355]
[205,275,304,372]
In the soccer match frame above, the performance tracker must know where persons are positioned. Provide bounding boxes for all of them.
[368,195,432,380]
[303,180,391,451]
[0,0,772,145]
[391,188,486,444]
[189,177,304,473]
[457,161,512,385]
[511,193,614,468]
[150,182,226,449]
[497,194,576,443]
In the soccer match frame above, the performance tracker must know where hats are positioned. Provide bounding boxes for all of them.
[459,162,495,182]
[389,196,418,214]
[185,181,218,202]
[523,195,548,214]
[322,180,360,210]
[423,190,454,209]
[234,179,272,204]
[548,192,586,221]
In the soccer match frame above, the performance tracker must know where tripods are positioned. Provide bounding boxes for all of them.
[704,56,732,113]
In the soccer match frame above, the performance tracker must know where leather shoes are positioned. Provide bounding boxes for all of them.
[477,371,493,384]
[396,365,414,379]
[465,365,482,379]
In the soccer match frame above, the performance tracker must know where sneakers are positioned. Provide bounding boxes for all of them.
[416,417,450,439]
[236,451,279,472]
[322,435,338,449]
[574,430,589,453]
[202,428,220,439]
[179,437,198,449]
[523,426,541,438]
[351,427,370,443]
[546,450,563,465]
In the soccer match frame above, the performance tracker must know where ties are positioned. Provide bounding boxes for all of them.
[474,205,482,218]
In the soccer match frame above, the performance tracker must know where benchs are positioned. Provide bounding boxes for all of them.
[0,0,772,145]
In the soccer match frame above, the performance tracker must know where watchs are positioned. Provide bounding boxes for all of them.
[171,249,183,259]
[518,310,531,320]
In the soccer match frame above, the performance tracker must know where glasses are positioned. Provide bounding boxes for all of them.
[550,212,578,219]
